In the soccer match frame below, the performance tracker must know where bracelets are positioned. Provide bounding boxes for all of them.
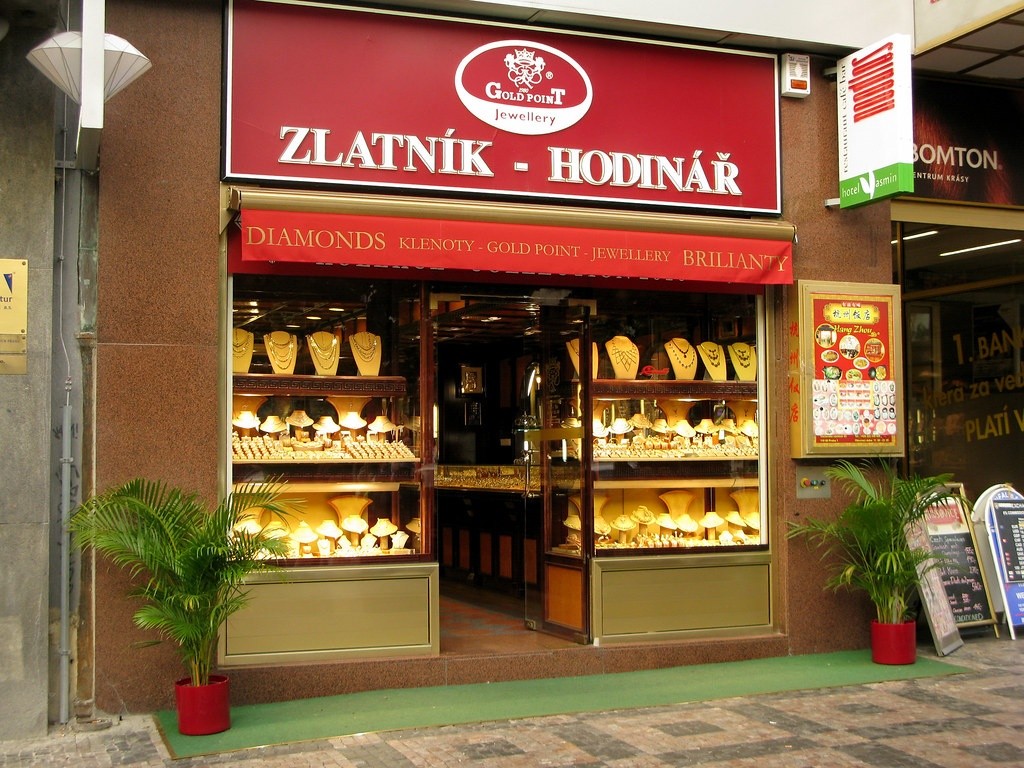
[438,473,541,489]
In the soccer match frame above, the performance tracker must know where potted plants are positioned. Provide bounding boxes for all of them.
[782,443,976,665]
[61,473,307,736]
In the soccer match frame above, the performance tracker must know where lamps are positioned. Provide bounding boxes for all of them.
[25,0,152,171]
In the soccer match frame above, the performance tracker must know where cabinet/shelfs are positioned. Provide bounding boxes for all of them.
[231,282,434,568]
[539,306,769,648]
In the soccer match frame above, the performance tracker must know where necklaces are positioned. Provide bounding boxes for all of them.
[233,331,252,359]
[308,333,337,370]
[234,414,388,431]
[239,519,421,554]
[611,339,637,372]
[570,341,579,356]
[701,346,720,367]
[267,332,294,370]
[565,419,758,449]
[564,508,760,535]
[351,331,377,362]
[731,345,751,368]
[670,340,695,369]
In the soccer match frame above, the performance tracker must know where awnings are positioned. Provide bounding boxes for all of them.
[228,187,797,286]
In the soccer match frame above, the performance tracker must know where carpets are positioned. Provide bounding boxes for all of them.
[149,649,979,760]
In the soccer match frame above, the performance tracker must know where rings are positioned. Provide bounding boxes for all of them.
[597,534,708,548]
[232,433,413,460]
[593,446,759,456]
